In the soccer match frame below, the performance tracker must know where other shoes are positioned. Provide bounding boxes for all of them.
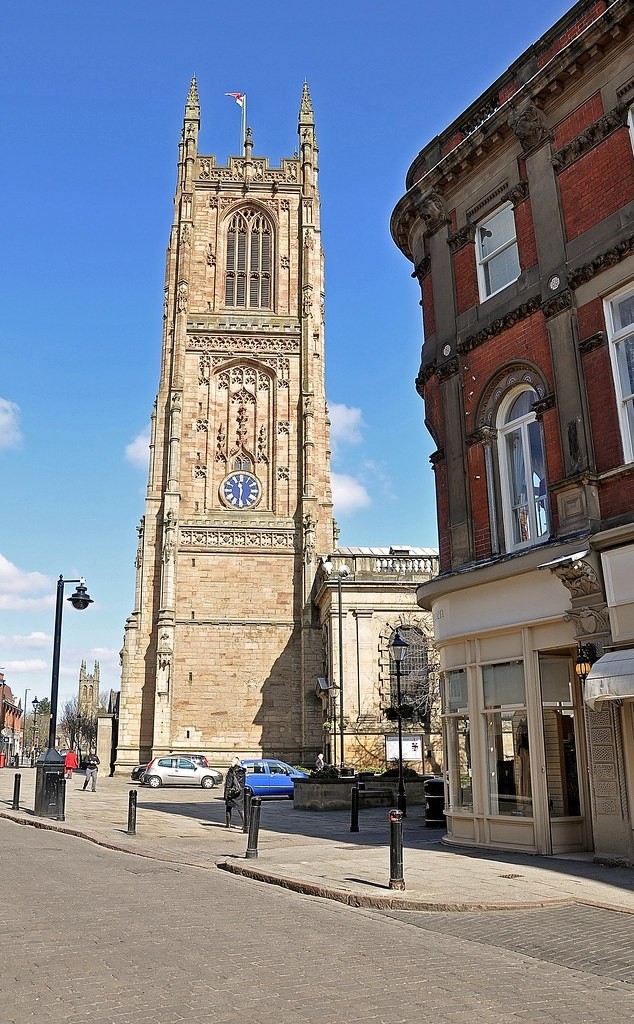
[92,789,96,792]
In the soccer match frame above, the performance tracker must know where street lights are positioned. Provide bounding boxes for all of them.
[30,696,41,768]
[321,561,351,765]
[21,688,31,765]
[34,574,95,818]
[383,629,410,817]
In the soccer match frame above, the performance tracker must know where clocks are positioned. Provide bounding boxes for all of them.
[219,471,262,510]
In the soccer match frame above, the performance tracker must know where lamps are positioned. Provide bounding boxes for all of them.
[575,643,597,684]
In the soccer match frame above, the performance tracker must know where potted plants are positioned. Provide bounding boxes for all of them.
[323,722,330,732]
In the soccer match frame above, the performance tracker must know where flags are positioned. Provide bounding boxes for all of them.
[224,92,244,107]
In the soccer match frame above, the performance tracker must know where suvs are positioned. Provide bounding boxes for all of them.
[131,753,211,785]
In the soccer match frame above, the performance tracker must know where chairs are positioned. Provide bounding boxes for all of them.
[254,765,266,773]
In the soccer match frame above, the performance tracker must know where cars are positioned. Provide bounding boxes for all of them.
[144,756,223,789]
[239,759,310,800]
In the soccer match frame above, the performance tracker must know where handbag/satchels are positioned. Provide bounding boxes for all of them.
[227,769,243,800]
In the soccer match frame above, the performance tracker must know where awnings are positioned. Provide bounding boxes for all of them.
[584,648,634,712]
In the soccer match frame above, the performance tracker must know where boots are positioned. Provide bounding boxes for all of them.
[239,811,245,830]
[224,811,232,828]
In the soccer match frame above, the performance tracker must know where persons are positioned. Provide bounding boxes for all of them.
[316,754,328,772]
[272,767,282,774]
[83,746,101,792]
[14,753,20,769]
[65,750,79,779]
[539,478,545,508]
[224,756,247,830]
